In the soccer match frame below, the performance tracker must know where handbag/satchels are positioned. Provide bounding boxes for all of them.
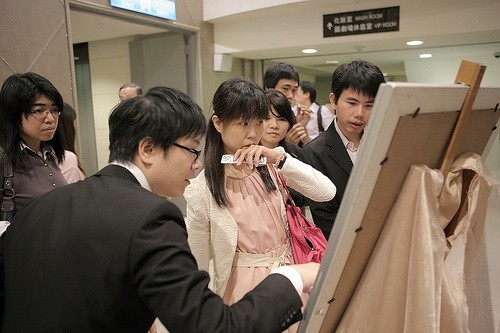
[268,162,328,264]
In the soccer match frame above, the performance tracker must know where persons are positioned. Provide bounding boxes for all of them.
[291,81,334,141]
[302,61,385,241]
[0,86,320,333]
[150,77,337,333]
[263,63,313,149]
[0,72,69,222]
[258,88,316,226]
[119,82,143,102]
[40,103,86,184]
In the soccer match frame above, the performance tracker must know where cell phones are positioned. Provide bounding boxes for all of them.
[221,154,267,166]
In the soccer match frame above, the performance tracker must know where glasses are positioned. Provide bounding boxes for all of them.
[26,109,62,117]
[171,143,202,162]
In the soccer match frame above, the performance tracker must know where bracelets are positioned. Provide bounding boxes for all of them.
[273,148,285,168]
